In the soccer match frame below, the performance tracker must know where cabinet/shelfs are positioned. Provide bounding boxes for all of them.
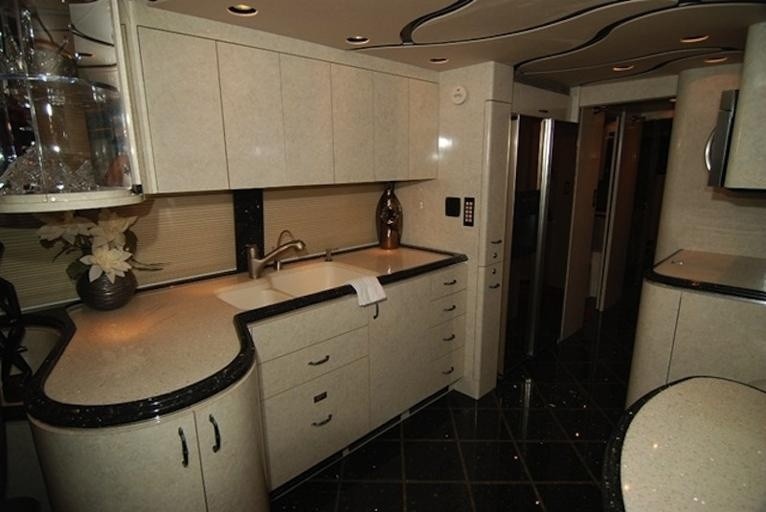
[626,280,766,411]
[26,361,270,512]
[431,263,467,401]
[246,296,370,502]
[279,53,374,187]
[119,24,285,194]
[373,70,440,185]
[0,0,146,213]
[369,276,431,438]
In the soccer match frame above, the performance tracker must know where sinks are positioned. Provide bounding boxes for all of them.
[215,282,293,312]
[266,260,382,299]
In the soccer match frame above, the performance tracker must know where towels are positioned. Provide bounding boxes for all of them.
[347,275,387,308]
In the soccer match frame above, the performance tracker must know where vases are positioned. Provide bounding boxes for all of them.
[76,263,138,314]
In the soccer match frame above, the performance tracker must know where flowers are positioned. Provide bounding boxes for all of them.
[33,205,167,281]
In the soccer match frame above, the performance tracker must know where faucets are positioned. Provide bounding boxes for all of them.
[246,239,305,280]
[276,229,296,269]
